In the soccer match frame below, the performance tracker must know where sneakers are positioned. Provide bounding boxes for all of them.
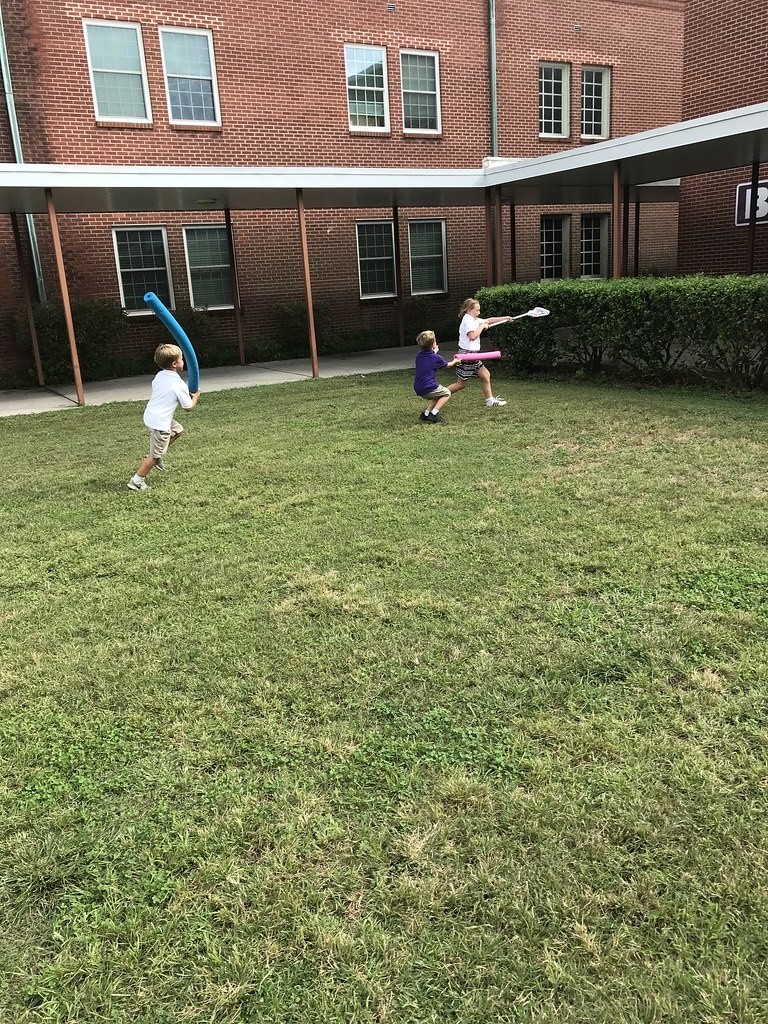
[127,477,151,491]
[146,454,165,471]
[427,412,445,423]
[418,412,429,421]
[486,394,507,407]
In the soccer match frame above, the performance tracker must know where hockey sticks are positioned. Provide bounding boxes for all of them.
[484,306,551,331]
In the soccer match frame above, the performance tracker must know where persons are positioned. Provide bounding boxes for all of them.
[127,342,201,491]
[428,298,514,407]
[414,330,462,423]
